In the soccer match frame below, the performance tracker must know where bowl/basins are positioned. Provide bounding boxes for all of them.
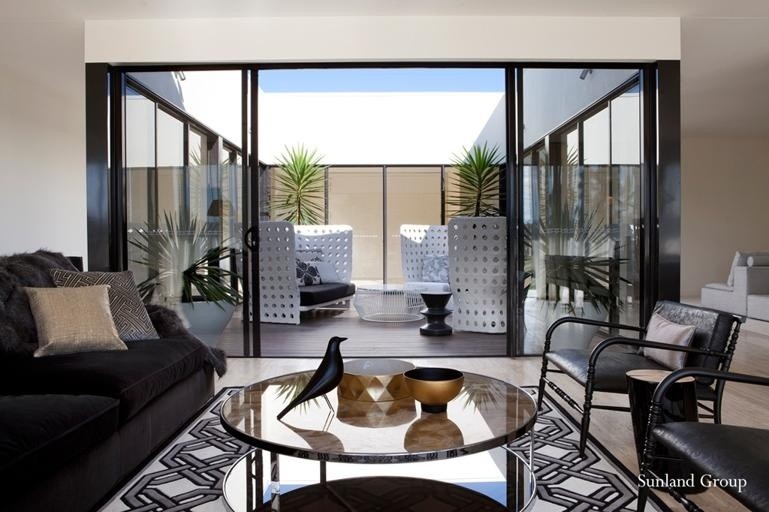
[337,357,416,402]
[403,366,463,413]
[403,412,464,453]
[335,397,418,428]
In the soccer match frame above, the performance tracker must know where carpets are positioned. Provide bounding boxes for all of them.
[105,377,673,509]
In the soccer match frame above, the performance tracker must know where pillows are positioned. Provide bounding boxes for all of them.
[298,246,322,262]
[19,286,131,358]
[47,261,161,344]
[421,255,450,282]
[295,258,321,287]
[312,261,341,284]
[643,312,692,370]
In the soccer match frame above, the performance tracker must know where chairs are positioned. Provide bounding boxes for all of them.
[535,293,748,462]
[631,362,768,512]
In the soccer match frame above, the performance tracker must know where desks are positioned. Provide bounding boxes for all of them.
[353,279,433,322]
[217,360,543,509]
[623,364,709,494]
[420,289,454,336]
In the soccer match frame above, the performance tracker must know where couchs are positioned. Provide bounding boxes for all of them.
[292,242,355,324]
[746,255,769,325]
[2,241,220,509]
[697,249,750,316]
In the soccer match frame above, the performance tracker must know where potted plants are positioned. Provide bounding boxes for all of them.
[516,193,635,355]
[126,207,250,347]
